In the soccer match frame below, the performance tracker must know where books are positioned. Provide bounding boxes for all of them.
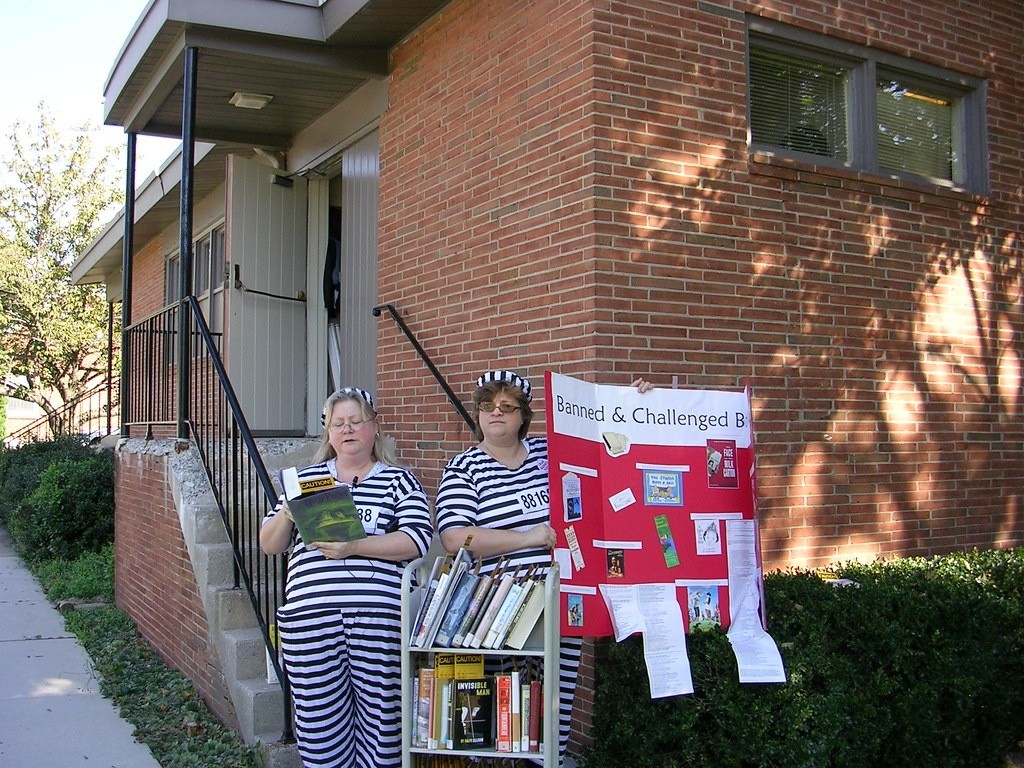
[412,653,544,755]
[281,466,367,551]
[408,547,545,650]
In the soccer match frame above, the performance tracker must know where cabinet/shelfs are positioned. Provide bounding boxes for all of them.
[401,559,560,768]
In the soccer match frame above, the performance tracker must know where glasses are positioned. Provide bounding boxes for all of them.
[328,417,374,432]
[475,402,521,413]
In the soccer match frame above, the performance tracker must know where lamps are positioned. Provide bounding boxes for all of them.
[228,92,274,110]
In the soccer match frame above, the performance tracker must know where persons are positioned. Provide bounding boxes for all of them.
[570,603,578,622]
[259,387,434,768]
[691,591,712,623]
[610,556,621,574]
[436,370,655,768]
